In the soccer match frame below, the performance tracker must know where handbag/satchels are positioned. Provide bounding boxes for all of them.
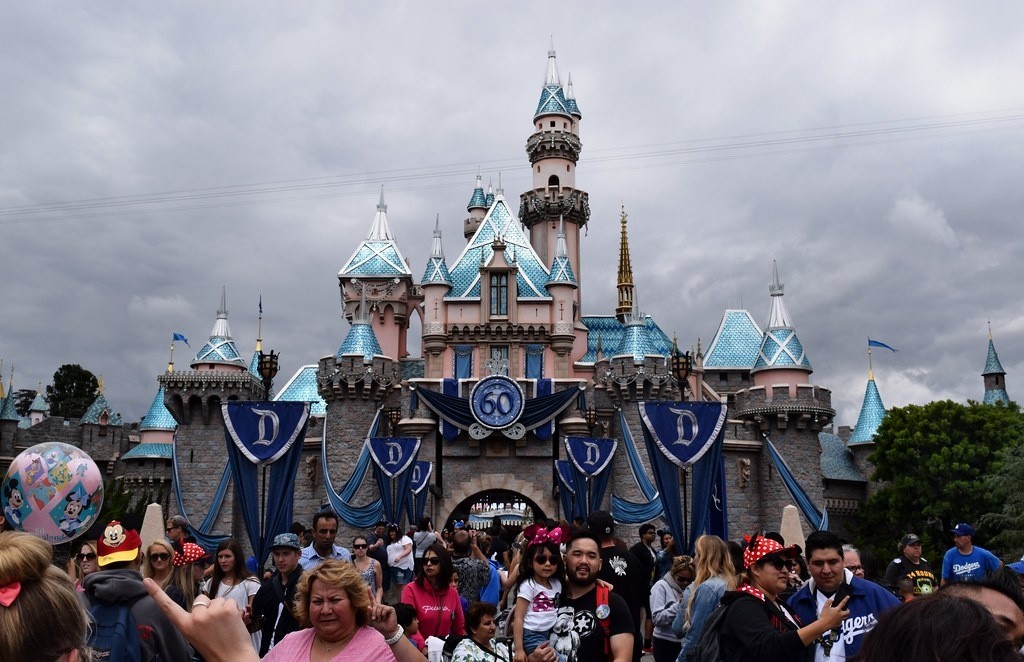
[424,635,445,662]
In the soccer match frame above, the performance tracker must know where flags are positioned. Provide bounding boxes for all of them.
[868,339,898,352]
[174,334,188,344]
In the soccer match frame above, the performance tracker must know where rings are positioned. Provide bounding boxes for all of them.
[192,602,207,608]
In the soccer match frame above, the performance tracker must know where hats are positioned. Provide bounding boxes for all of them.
[452,520,464,529]
[586,511,615,538]
[950,524,974,536]
[267,533,301,552]
[898,534,920,553]
[170,535,207,565]
[96,520,143,566]
[743,530,802,569]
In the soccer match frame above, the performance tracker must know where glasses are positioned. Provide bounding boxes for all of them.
[423,557,440,566]
[76,552,97,564]
[646,530,657,536]
[533,554,558,566]
[353,544,368,549]
[845,565,865,574]
[149,552,171,562]
[674,573,693,584]
[759,556,794,573]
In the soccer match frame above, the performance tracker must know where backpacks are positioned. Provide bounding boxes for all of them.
[684,596,763,662]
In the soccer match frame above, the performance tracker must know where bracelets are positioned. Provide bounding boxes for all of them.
[384,624,404,644]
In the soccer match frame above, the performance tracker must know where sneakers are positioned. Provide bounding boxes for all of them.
[642,645,653,655]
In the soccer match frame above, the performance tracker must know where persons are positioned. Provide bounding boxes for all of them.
[0,510,1024,662]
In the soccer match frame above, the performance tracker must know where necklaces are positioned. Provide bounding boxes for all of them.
[226,577,232,583]
[316,641,349,652]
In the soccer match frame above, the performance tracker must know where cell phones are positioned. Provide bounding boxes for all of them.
[831,582,855,611]
